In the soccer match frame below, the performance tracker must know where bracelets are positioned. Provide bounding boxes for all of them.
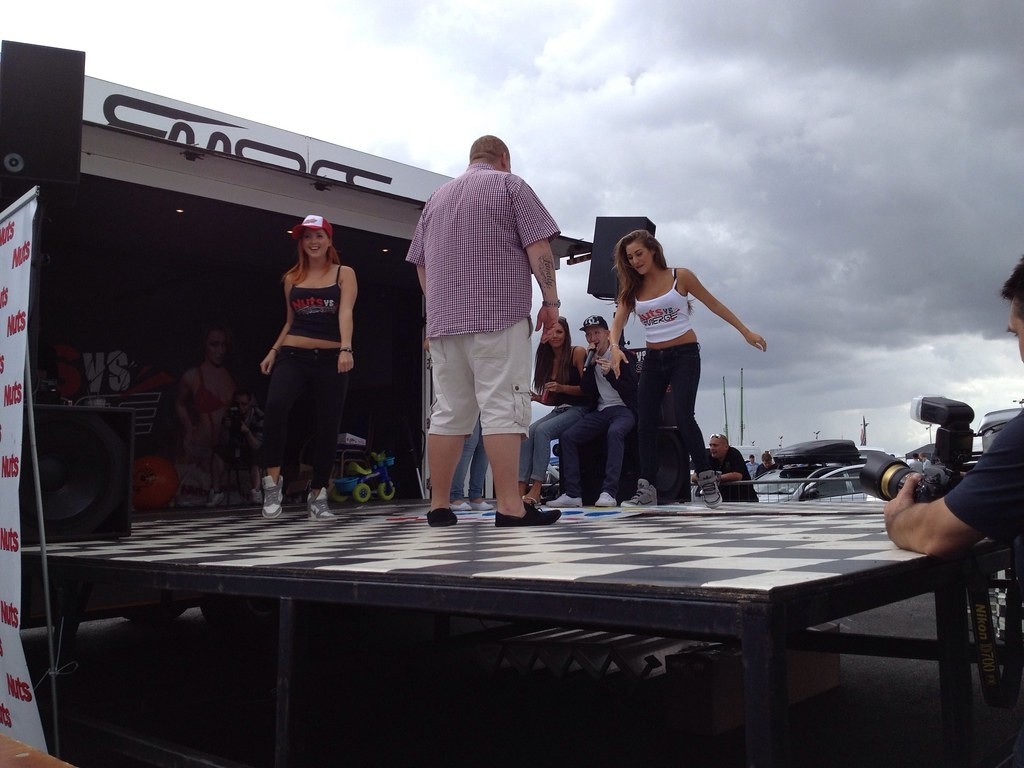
[609,344,619,351]
[271,345,280,352]
[340,346,352,352]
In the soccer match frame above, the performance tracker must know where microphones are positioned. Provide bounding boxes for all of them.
[583,345,597,372]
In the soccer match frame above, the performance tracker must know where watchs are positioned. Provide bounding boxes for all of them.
[542,300,562,307]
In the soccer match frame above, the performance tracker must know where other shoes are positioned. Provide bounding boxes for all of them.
[206,492,224,507]
[449,502,472,510]
[470,502,493,510]
[427,508,457,527]
[495,500,561,527]
[252,489,262,504]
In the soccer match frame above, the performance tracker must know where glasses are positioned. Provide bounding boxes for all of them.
[710,434,726,439]
[560,317,566,323]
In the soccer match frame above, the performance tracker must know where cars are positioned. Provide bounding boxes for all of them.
[753,438,880,504]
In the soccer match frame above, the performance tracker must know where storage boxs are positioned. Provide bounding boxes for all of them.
[335,431,367,445]
[665,620,843,736]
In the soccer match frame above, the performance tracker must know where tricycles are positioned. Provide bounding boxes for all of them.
[327,450,397,510]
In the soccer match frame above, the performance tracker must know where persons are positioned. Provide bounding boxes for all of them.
[518,315,636,509]
[175,323,243,508]
[451,413,494,511]
[204,390,264,506]
[890,451,932,471]
[404,135,561,527]
[608,229,773,509]
[883,255,1024,692]
[260,215,357,522]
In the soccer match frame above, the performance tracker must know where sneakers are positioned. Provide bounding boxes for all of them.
[262,475,283,518]
[697,471,722,509]
[307,487,337,522]
[546,494,582,508]
[621,479,657,508]
[595,492,617,506]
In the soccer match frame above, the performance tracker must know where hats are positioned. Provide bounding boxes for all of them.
[292,215,332,239]
[579,315,608,330]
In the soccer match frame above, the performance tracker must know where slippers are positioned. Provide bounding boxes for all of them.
[524,497,541,506]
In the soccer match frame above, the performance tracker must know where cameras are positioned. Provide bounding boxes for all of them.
[859,395,975,503]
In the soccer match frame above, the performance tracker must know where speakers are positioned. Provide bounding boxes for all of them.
[587,216,656,297]
[19,404,137,543]
